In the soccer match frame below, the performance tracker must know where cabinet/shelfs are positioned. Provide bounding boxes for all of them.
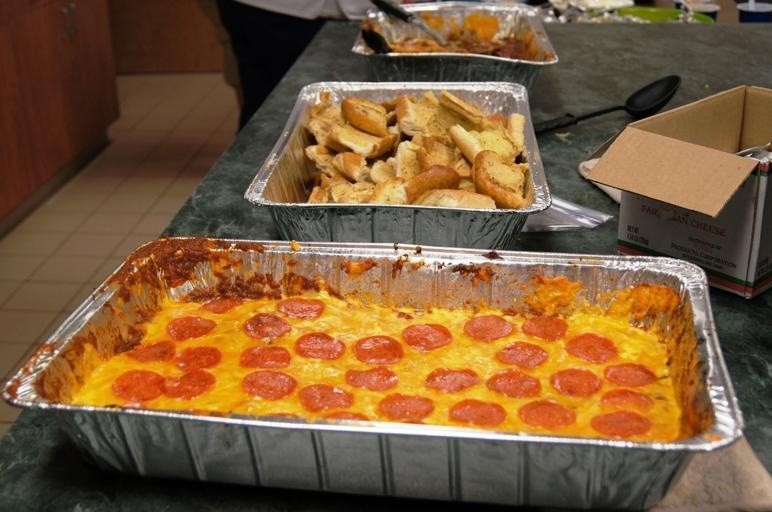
[0,0,125,241]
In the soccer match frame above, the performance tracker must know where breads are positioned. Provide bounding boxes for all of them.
[302,89,534,210]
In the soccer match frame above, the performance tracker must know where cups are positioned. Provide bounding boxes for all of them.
[682,1,772,24]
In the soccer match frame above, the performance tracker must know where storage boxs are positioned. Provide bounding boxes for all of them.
[578,80,772,305]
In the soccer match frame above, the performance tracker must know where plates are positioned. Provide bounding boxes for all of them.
[572,7,716,25]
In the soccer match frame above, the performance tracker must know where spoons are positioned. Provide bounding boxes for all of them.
[533,70,682,135]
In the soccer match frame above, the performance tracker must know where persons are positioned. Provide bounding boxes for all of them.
[212,0,406,141]
